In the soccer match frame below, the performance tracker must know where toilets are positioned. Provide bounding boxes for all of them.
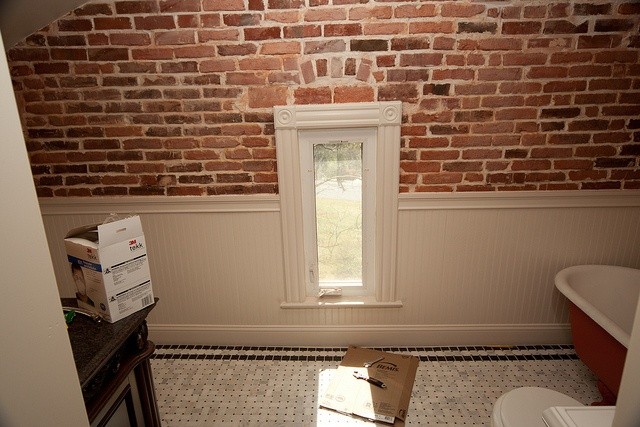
[490,386,584,427]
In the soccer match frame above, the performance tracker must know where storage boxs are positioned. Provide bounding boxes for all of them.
[65,215,156,323]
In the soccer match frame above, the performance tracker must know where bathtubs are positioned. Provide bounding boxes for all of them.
[551,263,639,406]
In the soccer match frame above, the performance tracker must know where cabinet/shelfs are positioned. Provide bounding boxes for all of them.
[58,295,162,427]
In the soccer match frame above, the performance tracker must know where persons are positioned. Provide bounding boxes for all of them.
[71,264,95,307]
